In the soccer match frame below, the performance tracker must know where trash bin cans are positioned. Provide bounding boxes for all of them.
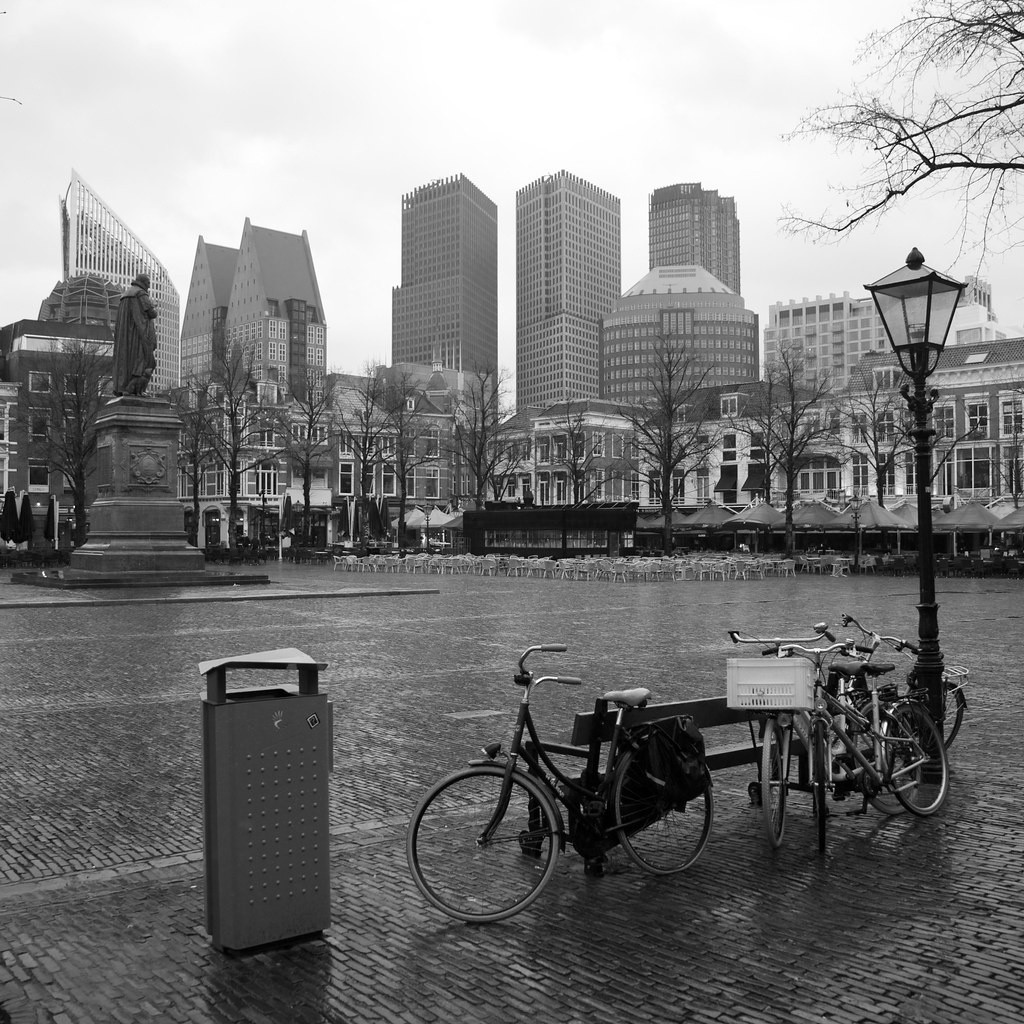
[194,645,337,961]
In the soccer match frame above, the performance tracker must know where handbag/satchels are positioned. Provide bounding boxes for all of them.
[616,713,712,811]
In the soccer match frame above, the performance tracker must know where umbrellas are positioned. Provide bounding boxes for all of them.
[338,494,392,548]
[0,488,54,550]
[391,505,466,548]
[279,493,295,539]
[635,497,1024,557]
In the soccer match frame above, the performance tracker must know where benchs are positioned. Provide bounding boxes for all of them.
[518,694,803,874]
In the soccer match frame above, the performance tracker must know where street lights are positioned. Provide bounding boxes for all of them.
[864,245,972,783]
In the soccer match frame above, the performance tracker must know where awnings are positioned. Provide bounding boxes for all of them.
[714,472,764,492]
[256,508,279,514]
[388,507,411,518]
[297,508,329,514]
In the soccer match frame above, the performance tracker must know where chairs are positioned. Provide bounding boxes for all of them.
[205,543,1024,584]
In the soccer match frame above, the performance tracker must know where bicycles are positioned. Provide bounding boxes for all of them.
[724,612,974,853]
[406,640,715,923]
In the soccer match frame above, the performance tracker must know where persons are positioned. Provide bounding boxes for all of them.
[240,533,261,562]
[112,273,158,397]
